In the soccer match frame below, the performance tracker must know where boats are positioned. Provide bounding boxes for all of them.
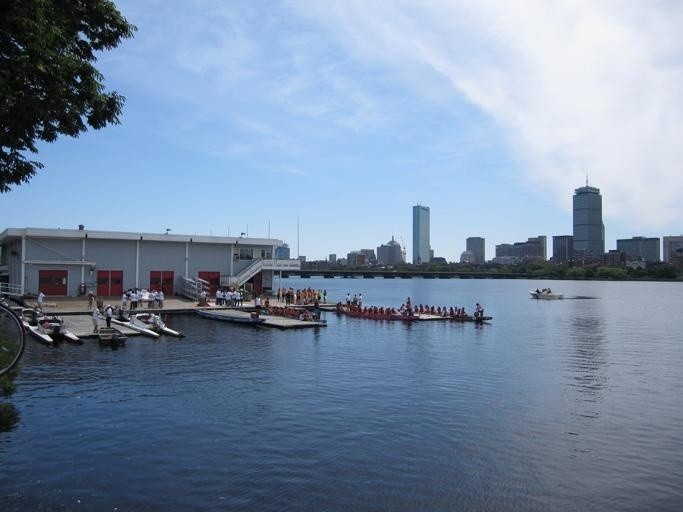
[196,309,265,324]
[530,291,562,299]
[414,306,492,321]
[348,311,419,321]
[38,320,66,335]
[21,307,44,322]
[261,306,327,326]
[130,313,159,330]
[97,327,127,350]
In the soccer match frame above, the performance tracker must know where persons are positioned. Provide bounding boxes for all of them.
[35,290,45,311]
[87,294,93,310]
[397,296,414,317]
[91,305,102,333]
[352,293,357,304]
[357,293,363,306]
[199,283,326,307]
[345,292,350,306]
[120,288,172,309]
[102,304,112,326]
[475,301,484,316]
